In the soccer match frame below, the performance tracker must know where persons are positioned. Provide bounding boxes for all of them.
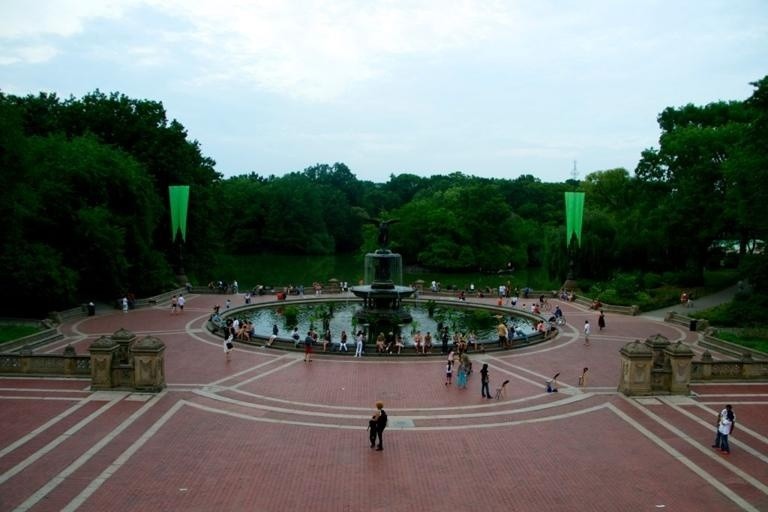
[186,280,192,293]
[687,292,694,309]
[712,405,736,448]
[376,402,387,451]
[719,410,733,454]
[178,294,184,312]
[408,281,605,398]
[738,279,743,290]
[208,280,367,364]
[122,296,129,311]
[681,291,689,308]
[171,294,177,314]
[367,415,377,448]
[376,331,405,357]
